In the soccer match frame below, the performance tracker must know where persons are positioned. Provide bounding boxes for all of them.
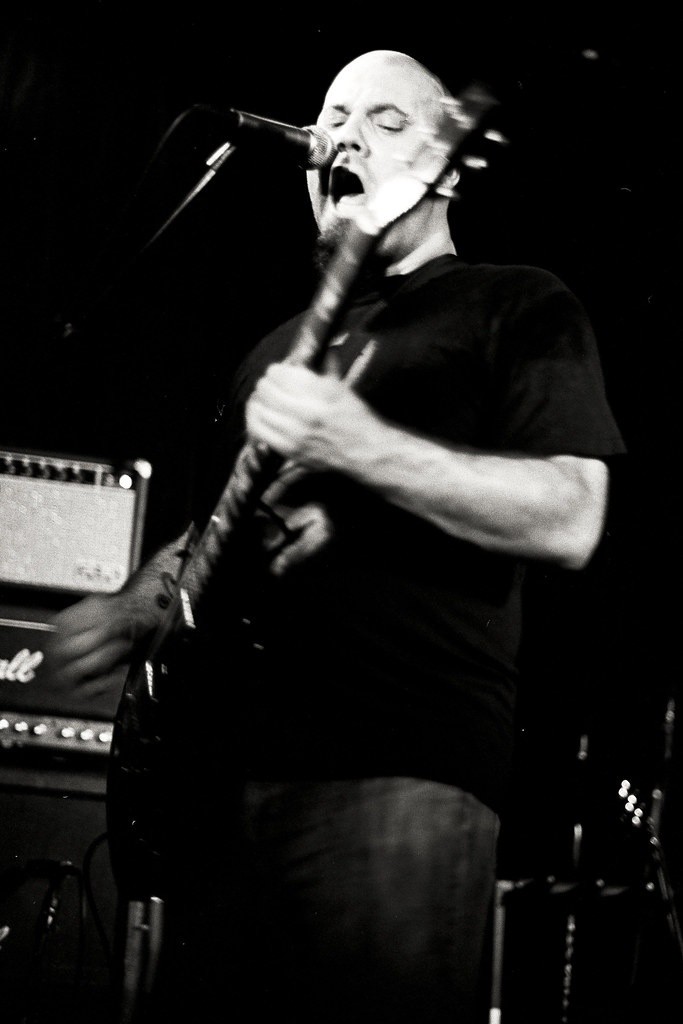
[48,48,630,1024]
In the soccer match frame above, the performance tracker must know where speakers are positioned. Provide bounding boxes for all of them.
[1,450,152,1023]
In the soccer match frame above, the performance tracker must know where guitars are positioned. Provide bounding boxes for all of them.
[105,78,504,901]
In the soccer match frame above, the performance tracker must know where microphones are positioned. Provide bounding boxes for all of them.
[199,102,334,170]
